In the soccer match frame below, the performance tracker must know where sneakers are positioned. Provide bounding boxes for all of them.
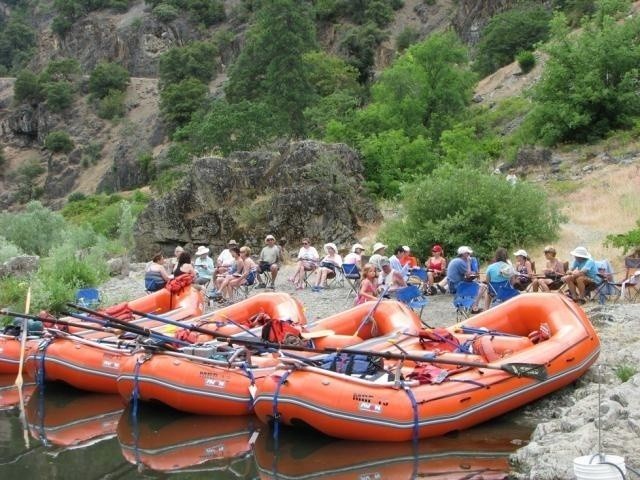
[255,283,266,289]
[267,284,274,289]
[218,301,234,308]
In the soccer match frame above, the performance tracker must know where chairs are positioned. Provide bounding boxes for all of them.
[77,290,99,316]
[453,281,479,323]
[397,286,431,328]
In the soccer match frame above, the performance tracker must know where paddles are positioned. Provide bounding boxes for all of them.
[324,336,547,381]
[131,309,260,348]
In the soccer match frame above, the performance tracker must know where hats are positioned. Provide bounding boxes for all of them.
[265,235,276,244]
[378,257,390,265]
[570,246,591,259]
[458,246,473,254]
[228,240,239,244]
[432,246,441,252]
[513,250,527,257]
[195,246,209,255]
[324,243,338,255]
[372,242,388,255]
[402,246,410,252]
[353,244,365,251]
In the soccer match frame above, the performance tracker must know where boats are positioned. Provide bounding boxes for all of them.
[256,293,601,442]
[0,283,205,373]
[25,290,307,425]
[116,298,423,421]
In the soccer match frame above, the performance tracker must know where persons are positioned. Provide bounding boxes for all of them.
[290,237,446,306]
[144,233,282,309]
[432,244,602,313]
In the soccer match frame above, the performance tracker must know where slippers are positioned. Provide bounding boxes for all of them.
[207,292,222,298]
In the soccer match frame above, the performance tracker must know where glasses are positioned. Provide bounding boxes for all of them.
[302,243,307,245]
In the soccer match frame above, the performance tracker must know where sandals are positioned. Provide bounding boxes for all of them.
[218,298,227,303]
[213,296,222,302]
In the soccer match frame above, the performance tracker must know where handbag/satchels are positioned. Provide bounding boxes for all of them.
[520,269,529,283]
